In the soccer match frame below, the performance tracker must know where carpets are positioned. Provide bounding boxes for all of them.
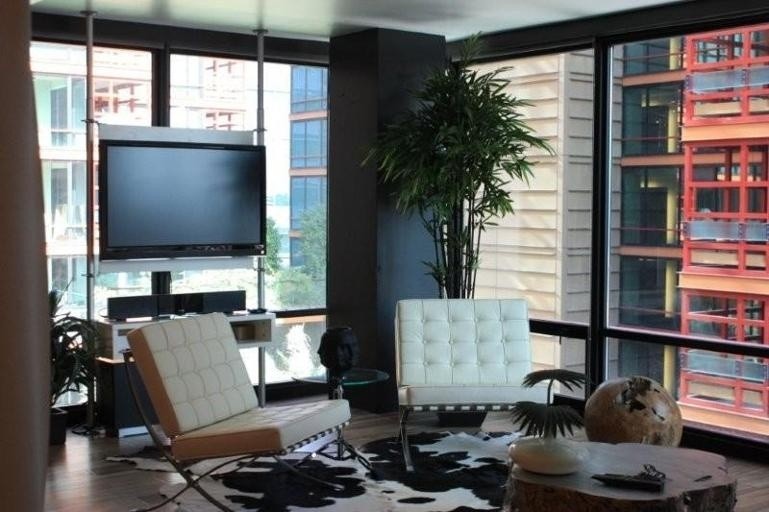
[103,432,524,511]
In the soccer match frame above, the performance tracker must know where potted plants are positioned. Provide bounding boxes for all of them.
[47,279,105,446]
[358,27,559,427]
[507,368,597,475]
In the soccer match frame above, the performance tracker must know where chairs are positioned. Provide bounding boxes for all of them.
[125,311,376,512]
[391,297,554,472]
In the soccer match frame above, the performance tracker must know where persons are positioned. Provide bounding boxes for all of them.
[318,327,361,379]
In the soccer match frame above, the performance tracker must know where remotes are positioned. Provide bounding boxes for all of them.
[592,471,665,491]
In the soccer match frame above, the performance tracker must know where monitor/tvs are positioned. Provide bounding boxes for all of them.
[98,140,267,259]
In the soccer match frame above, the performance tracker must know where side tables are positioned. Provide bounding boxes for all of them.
[290,366,390,473]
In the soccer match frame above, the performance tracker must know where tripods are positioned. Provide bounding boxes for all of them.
[292,384,374,471]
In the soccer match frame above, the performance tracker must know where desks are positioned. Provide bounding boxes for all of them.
[73,308,276,440]
[502,441,737,512]
